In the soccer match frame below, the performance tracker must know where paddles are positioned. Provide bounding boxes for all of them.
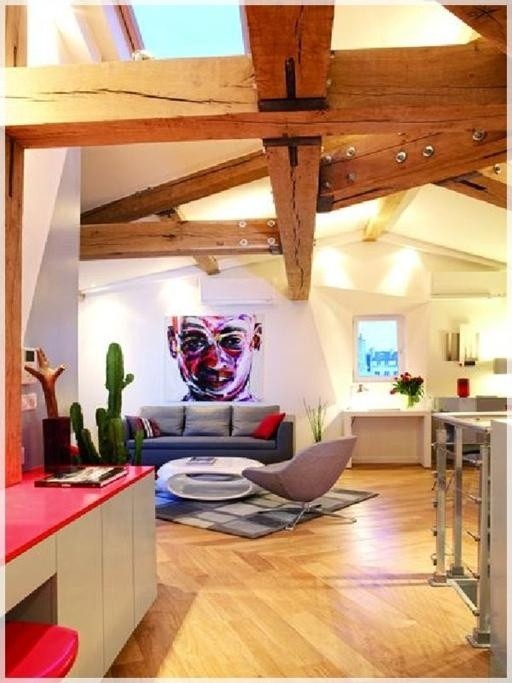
[156,477,381,541]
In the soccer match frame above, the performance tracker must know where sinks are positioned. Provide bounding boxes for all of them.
[122,404,297,473]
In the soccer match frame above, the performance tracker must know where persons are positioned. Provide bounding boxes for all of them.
[163,314,262,403]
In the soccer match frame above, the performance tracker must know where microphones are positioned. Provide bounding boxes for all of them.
[5,618,81,678]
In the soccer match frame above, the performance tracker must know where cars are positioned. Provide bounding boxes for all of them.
[44,417,71,473]
[458,378,469,397]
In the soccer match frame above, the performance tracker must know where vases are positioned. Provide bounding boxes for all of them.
[390,372,425,405]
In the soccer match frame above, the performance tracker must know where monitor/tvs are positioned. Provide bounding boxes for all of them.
[33,463,131,488]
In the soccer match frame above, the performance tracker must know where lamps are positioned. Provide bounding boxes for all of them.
[242,434,358,532]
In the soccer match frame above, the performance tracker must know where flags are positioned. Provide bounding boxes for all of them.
[406,394,416,410]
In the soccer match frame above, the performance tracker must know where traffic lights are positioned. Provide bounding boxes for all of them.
[125,414,161,439]
[251,411,285,439]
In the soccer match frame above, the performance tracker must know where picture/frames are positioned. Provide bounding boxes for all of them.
[343,407,432,470]
[5,465,160,678]
